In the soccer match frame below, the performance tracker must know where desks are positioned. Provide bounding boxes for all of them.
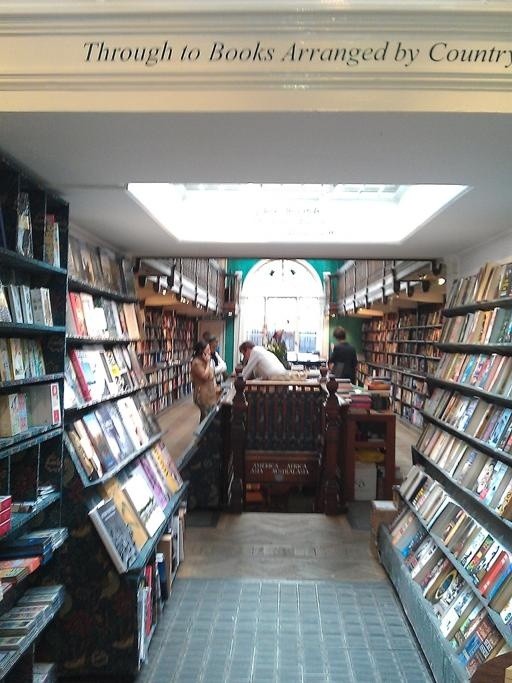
[287,351,326,369]
[222,373,396,504]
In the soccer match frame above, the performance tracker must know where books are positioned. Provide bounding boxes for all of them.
[398,423,511,535]
[33,663,56,678]
[447,266,510,307]
[334,376,372,413]
[2,338,45,379]
[2,383,61,438]
[64,392,162,482]
[88,440,183,574]
[64,345,147,410]
[67,293,140,338]
[68,236,134,296]
[361,310,443,372]
[387,504,511,679]
[137,507,186,659]
[2,192,60,268]
[424,353,512,447]
[2,284,53,327]
[2,481,56,534]
[139,312,196,415]
[439,307,510,343]
[2,585,65,677]
[368,368,426,429]
[2,528,71,595]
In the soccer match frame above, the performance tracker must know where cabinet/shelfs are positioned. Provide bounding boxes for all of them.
[0,152,223,683]
[354,256,512,683]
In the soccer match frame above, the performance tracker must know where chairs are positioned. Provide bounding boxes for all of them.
[233,376,342,515]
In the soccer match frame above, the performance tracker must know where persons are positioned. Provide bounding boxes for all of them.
[327,324,357,384]
[239,340,288,381]
[192,330,227,422]
